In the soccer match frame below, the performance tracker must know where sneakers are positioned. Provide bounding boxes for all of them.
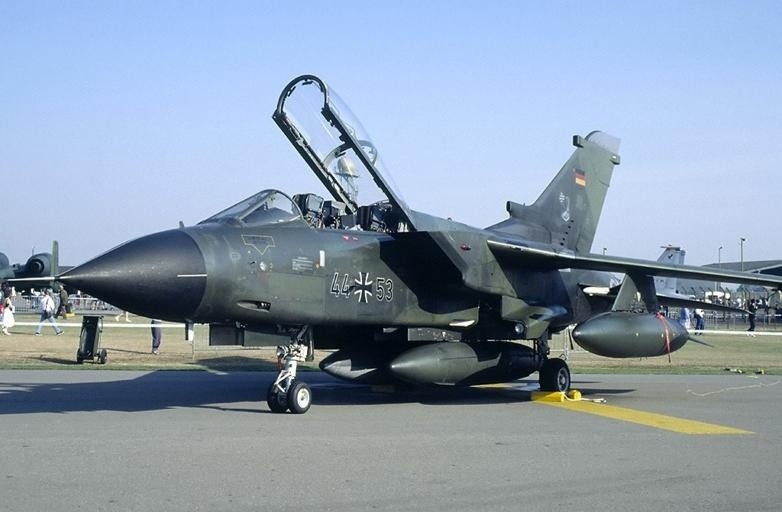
[34,332,40,336]
[152,351,159,355]
[57,330,64,336]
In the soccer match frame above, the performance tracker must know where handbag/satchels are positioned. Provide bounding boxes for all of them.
[44,310,55,320]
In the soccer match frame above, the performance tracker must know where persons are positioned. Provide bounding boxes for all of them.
[694,307,705,336]
[115,309,133,323]
[151,318,161,356]
[0,279,69,336]
[747,298,758,332]
[679,307,690,330]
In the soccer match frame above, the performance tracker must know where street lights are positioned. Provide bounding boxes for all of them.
[740,237,745,307]
[718,247,723,299]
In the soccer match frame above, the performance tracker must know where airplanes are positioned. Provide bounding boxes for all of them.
[5,75,781,413]
[0,241,75,298]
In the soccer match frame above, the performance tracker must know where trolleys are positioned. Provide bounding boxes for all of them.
[78,316,108,365]
